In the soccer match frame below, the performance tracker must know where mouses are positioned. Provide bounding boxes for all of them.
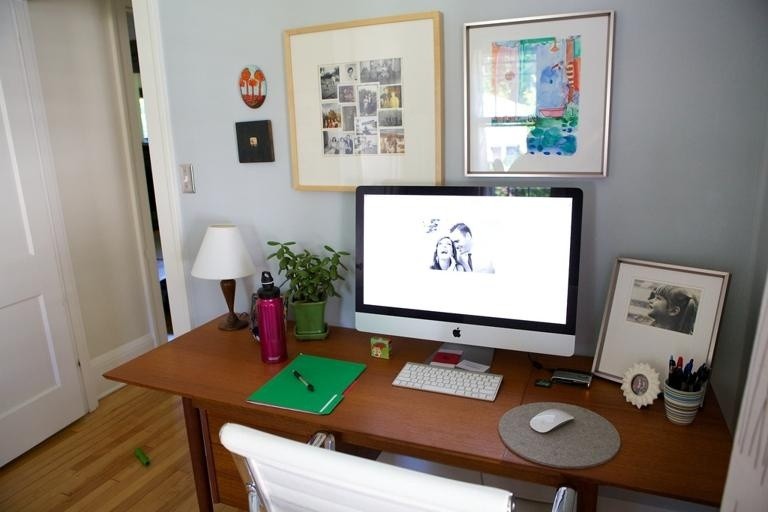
[530,409,574,433]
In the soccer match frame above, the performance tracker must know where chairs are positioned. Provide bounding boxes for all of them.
[213,416,583,511]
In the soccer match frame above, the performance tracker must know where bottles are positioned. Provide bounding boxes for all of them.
[253,271,289,364]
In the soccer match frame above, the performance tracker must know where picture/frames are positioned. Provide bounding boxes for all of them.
[283,10,445,195]
[462,8,614,178]
[589,254,729,407]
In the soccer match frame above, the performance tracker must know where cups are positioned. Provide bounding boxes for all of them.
[662,380,704,427]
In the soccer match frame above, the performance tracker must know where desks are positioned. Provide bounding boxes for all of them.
[99,312,738,512]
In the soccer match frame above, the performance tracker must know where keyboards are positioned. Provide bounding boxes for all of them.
[392,362,503,402]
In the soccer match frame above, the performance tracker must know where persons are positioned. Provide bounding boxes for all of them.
[427,236,459,272]
[449,222,495,274]
[313,56,406,155]
[643,283,700,333]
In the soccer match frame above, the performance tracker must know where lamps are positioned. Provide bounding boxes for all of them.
[191,224,257,330]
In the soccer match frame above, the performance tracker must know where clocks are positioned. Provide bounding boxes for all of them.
[620,362,660,411]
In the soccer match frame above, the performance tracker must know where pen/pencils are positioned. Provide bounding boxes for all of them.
[292,368,313,391]
[668,354,712,392]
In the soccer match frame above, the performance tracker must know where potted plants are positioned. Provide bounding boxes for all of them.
[266,235,350,342]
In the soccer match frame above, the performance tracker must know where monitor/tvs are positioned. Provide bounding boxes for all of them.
[355,186,583,374]
